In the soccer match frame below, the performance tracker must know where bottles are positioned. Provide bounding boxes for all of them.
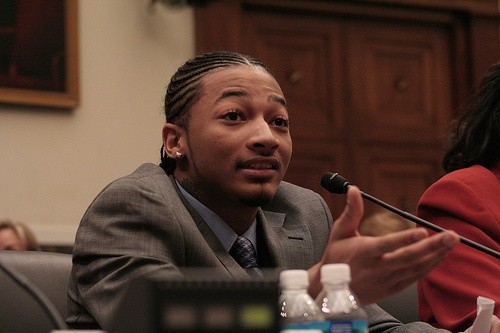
[279,269,326,333]
[314,263,368,333]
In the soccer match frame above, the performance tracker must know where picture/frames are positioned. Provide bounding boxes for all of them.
[0,0,81,109]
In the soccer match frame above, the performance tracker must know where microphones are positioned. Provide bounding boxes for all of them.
[320,173,500,258]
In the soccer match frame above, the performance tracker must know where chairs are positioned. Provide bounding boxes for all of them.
[0,251,78,333]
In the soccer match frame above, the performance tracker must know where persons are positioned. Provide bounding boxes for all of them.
[413,62,500,332]
[0,220,39,252]
[66,52,500,333]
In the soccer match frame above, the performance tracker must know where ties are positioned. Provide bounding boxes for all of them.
[234,237,259,270]
